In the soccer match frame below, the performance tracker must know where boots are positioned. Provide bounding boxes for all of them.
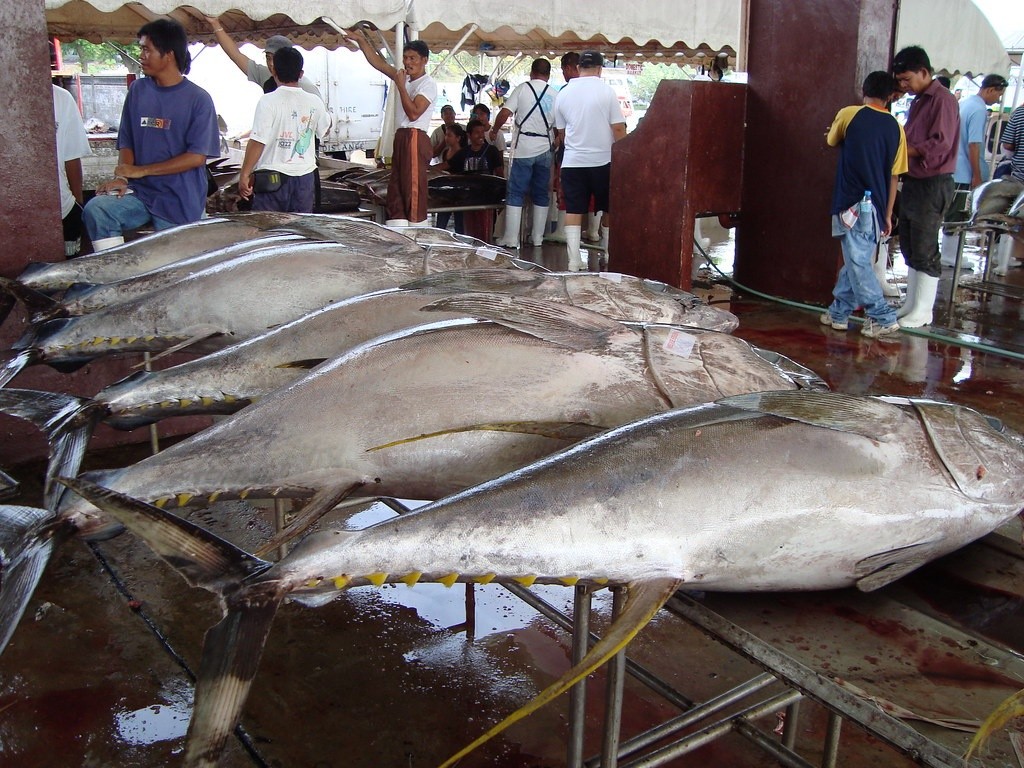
[528,205,550,246]
[893,266,941,328]
[992,234,1015,277]
[496,205,523,248]
[870,244,902,297]
[544,210,566,242]
[582,212,600,242]
[564,225,587,272]
[601,224,609,264]
[938,235,974,269]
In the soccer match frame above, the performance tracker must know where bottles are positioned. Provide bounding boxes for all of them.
[860,191,872,212]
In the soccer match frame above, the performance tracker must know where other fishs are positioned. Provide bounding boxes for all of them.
[0,209,553,393]
[0,291,834,654]
[48,386,1024,767]
[0,267,740,509]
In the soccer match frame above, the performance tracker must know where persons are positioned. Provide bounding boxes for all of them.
[341,28,438,227]
[83,19,221,254]
[53,85,93,261]
[374,78,513,241]
[874,74,1024,296]
[819,71,909,339]
[205,15,333,214]
[891,46,962,328]
[693,75,713,255]
[489,52,601,249]
[554,50,628,272]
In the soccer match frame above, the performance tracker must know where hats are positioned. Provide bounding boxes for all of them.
[579,49,606,68]
[261,35,293,54]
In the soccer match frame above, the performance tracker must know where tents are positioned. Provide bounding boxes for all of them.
[45,0,1010,269]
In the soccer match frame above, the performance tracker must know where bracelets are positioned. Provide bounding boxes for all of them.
[212,27,223,33]
[113,175,129,184]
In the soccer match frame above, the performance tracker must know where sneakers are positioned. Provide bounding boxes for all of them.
[820,310,849,329]
[860,317,900,339]
